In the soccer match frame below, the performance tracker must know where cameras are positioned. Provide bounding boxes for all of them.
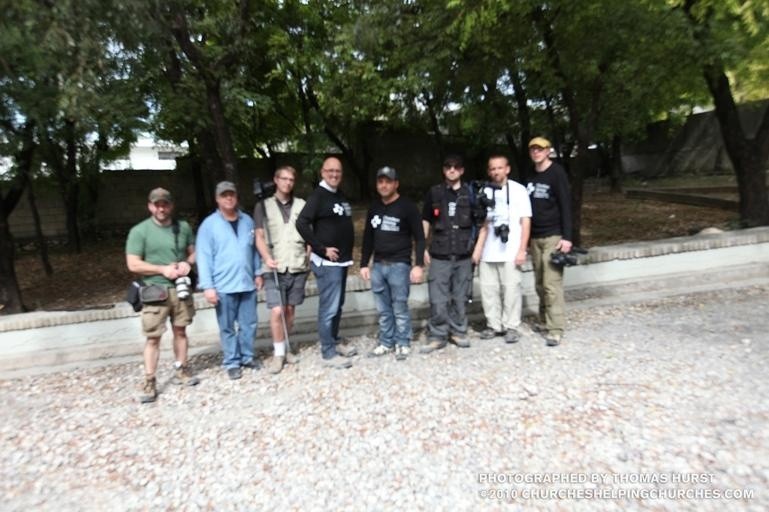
[495,224,509,242]
[549,253,577,271]
[171,272,197,300]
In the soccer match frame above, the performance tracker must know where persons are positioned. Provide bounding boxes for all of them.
[522,137,576,347]
[360,165,425,361]
[295,156,358,360]
[253,164,313,375]
[122,186,200,404]
[193,180,265,381]
[476,151,533,345]
[419,150,490,356]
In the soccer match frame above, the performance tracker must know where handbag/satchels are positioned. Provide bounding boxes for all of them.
[137,285,168,303]
[126,281,146,312]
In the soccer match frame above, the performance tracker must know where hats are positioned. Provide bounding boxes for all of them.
[148,188,171,203]
[444,156,464,167]
[376,166,399,180]
[528,137,551,148]
[216,181,237,195]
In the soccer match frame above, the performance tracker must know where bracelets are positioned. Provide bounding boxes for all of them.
[183,260,194,267]
[520,250,528,256]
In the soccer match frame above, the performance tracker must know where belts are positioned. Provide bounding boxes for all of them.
[433,254,469,261]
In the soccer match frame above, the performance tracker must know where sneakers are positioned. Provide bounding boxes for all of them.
[285,352,298,363]
[547,333,562,346]
[395,343,410,360]
[373,344,394,355]
[428,340,446,349]
[321,353,352,368]
[531,320,547,332]
[480,328,505,338]
[506,329,519,343]
[270,355,285,372]
[172,366,199,386]
[449,334,470,347]
[336,343,357,357]
[228,368,241,379]
[139,376,157,403]
[242,358,257,371]
